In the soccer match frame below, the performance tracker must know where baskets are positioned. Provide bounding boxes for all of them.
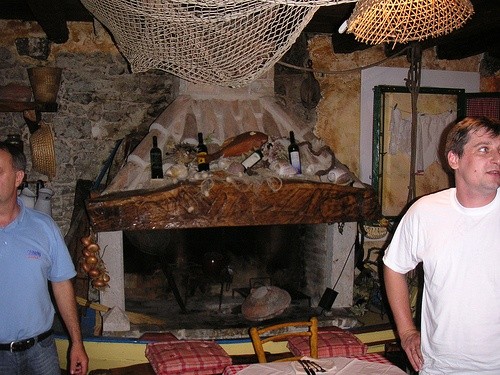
[30,120,57,181]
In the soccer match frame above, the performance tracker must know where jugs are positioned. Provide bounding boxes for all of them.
[18,181,36,210]
[34,179,55,218]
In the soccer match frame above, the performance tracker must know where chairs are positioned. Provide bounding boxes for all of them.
[249,317,317,362]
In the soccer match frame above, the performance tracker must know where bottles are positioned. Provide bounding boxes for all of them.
[288,131,302,176]
[197,132,211,172]
[150,136,164,179]
[242,143,274,172]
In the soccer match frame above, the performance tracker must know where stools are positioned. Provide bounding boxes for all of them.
[145,341,232,375]
[286,326,368,357]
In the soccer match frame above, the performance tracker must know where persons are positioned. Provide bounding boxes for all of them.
[382,116,500,375]
[0,144,88,375]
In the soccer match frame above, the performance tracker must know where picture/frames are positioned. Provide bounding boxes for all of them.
[373,85,467,222]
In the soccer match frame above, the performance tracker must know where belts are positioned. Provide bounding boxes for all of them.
[0,329,53,352]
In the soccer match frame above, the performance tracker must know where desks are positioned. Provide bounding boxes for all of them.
[222,353,407,375]
